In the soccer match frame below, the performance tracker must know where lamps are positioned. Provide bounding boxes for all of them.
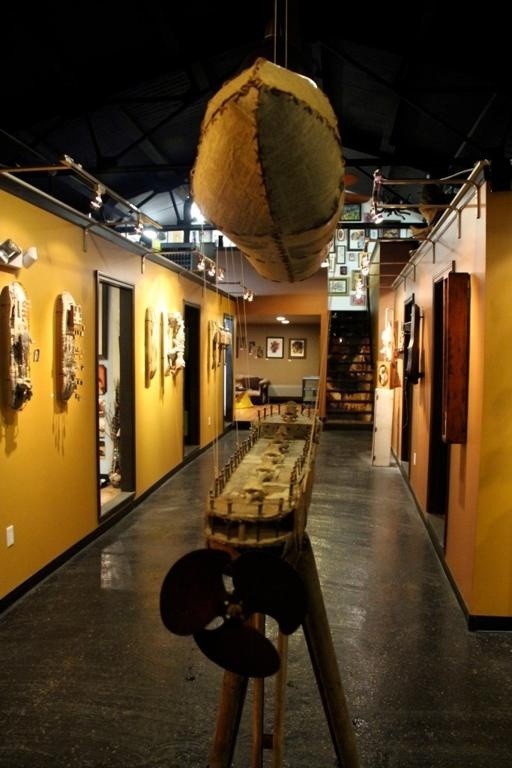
[237,283,257,302]
[192,246,226,282]
[1,239,38,272]
[66,159,163,236]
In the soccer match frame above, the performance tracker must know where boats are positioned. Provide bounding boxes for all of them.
[190,394,330,571]
[186,54,350,287]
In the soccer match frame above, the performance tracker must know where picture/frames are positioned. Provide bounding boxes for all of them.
[329,228,376,296]
[266,337,284,360]
[288,338,306,359]
[341,203,362,221]
[98,282,108,360]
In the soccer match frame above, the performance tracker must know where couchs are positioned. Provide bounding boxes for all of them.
[235,375,271,404]
